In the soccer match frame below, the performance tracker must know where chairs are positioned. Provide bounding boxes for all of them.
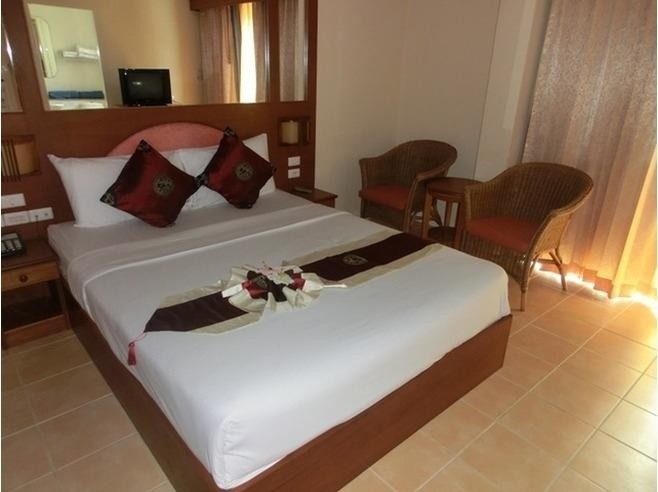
[360,139,457,233]
[462,161,593,311]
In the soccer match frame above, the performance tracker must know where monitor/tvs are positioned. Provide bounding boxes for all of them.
[119,68,172,106]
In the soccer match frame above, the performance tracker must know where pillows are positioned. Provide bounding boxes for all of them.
[46,126,276,228]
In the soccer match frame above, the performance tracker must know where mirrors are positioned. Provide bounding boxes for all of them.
[15,0,317,109]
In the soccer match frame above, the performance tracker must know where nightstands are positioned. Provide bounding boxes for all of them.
[1,240,69,350]
[291,184,340,211]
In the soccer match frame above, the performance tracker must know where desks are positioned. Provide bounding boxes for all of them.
[420,177,482,248]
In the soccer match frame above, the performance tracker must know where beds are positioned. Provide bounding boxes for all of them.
[7,110,515,492]
